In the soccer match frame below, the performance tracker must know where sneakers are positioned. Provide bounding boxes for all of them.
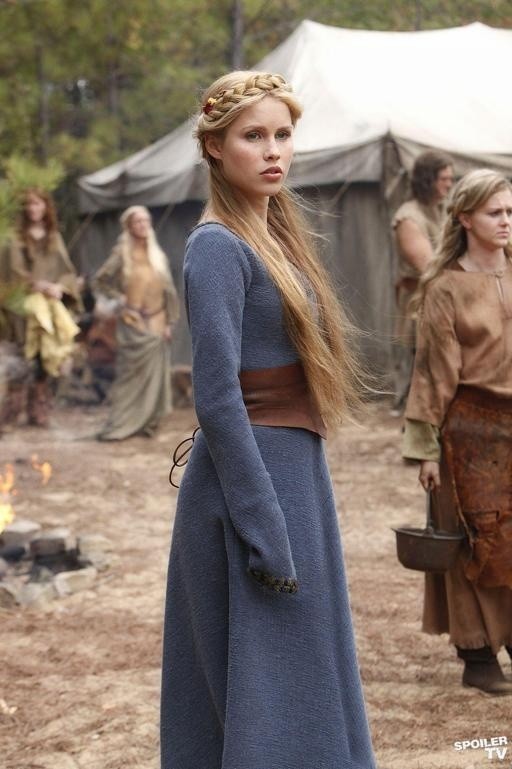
[460,654,512,697]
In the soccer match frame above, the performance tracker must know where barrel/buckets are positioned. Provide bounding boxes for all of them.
[391,478,467,571]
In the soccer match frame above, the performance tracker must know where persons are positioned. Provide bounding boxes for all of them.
[10,183,87,431]
[154,69,380,769]
[387,148,457,400]
[399,166,512,699]
[91,201,182,444]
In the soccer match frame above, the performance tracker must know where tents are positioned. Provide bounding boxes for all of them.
[66,17,510,412]
